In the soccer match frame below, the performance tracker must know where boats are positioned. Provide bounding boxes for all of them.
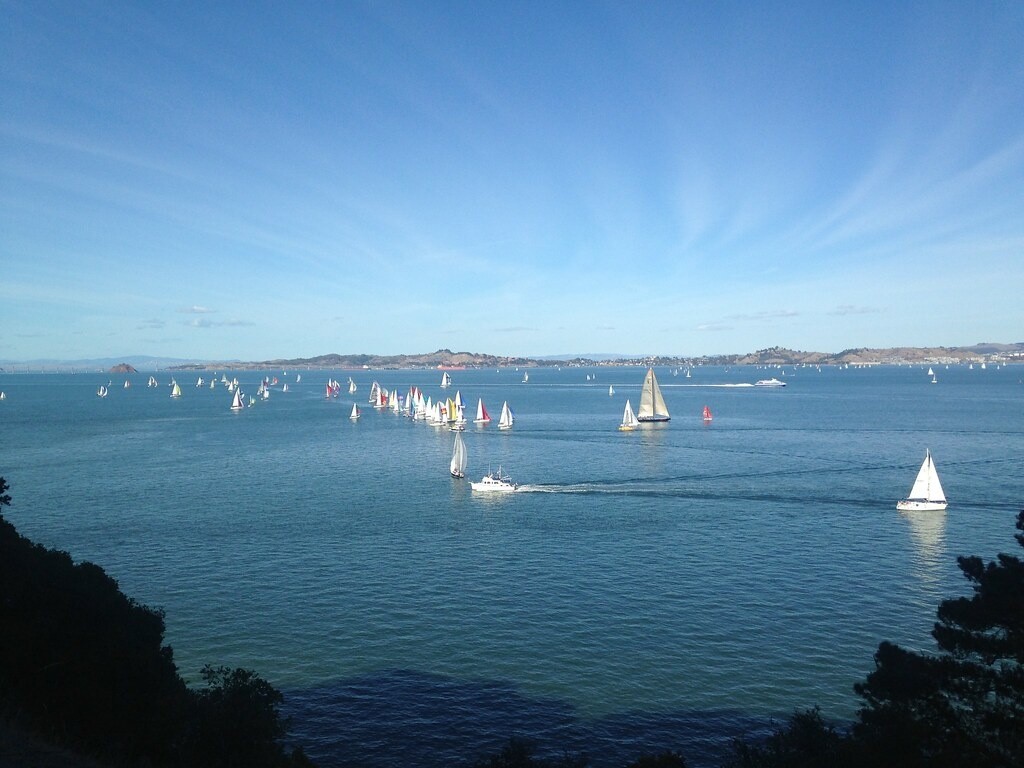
[467,461,518,492]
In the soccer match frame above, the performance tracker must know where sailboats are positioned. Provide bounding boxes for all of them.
[618,398,641,431]
[895,446,948,512]
[551,358,1009,396]
[0,356,537,435]
[702,404,713,420]
[448,430,468,479]
[635,366,671,423]
